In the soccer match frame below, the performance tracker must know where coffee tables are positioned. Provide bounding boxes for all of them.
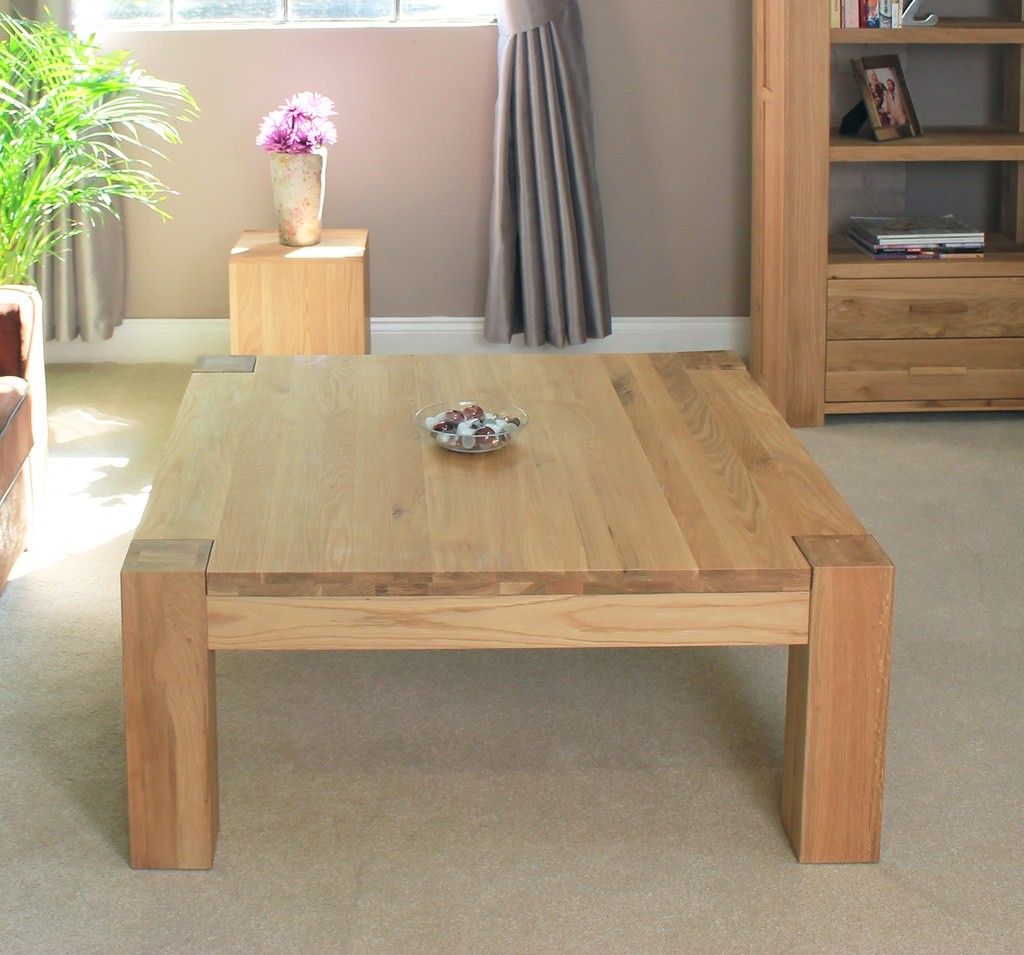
[118,351,896,870]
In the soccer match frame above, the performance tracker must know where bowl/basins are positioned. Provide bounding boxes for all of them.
[415,399,529,453]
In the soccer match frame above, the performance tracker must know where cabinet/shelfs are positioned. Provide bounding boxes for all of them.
[750,0,1024,428]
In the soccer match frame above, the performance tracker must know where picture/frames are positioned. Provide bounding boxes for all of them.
[851,54,922,142]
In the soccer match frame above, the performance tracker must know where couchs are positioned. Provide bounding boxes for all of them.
[0,284,45,594]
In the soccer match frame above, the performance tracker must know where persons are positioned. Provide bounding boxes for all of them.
[869,70,909,126]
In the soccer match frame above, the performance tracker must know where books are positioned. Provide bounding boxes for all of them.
[847,214,985,260]
[829,0,909,28]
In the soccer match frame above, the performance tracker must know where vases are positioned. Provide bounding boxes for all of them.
[269,149,327,248]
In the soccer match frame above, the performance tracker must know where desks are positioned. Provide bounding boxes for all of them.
[228,230,371,356]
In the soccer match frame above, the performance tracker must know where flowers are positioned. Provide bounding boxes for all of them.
[254,91,337,155]
